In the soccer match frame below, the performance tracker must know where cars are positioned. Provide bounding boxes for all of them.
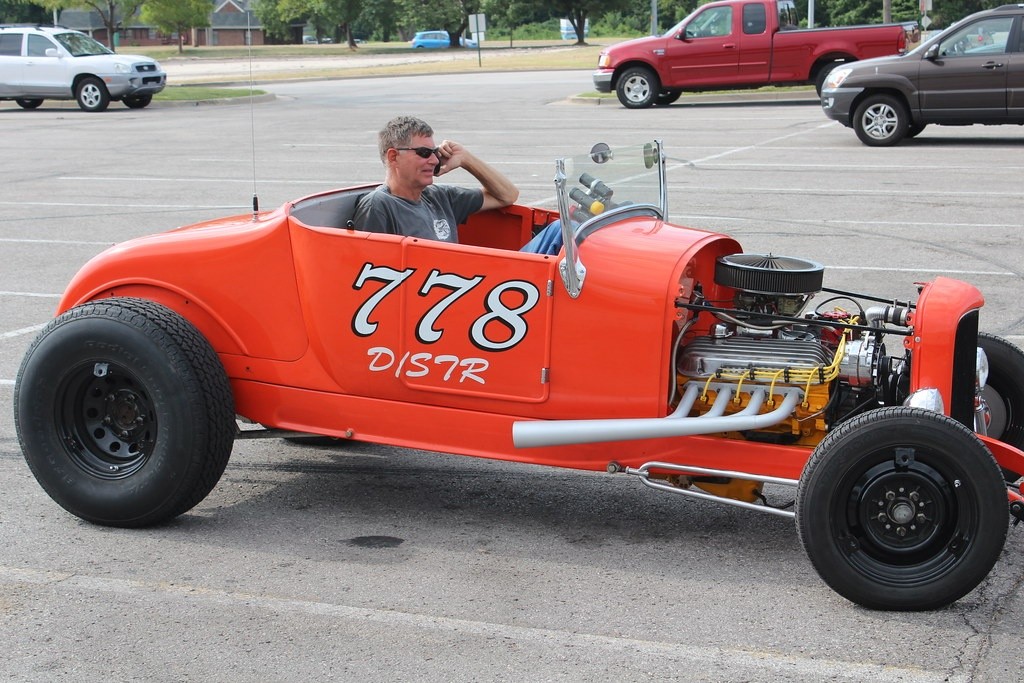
[411,31,478,48]
[13,140,1024,610]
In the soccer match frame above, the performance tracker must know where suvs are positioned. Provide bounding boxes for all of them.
[821,4,1024,146]
[0,24,167,112]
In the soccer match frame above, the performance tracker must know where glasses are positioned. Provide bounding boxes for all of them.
[384,147,440,159]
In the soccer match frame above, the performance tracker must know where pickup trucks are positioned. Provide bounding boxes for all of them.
[593,1,919,109]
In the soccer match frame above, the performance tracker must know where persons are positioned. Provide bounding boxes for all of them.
[909,26,919,42]
[354,116,584,257]
